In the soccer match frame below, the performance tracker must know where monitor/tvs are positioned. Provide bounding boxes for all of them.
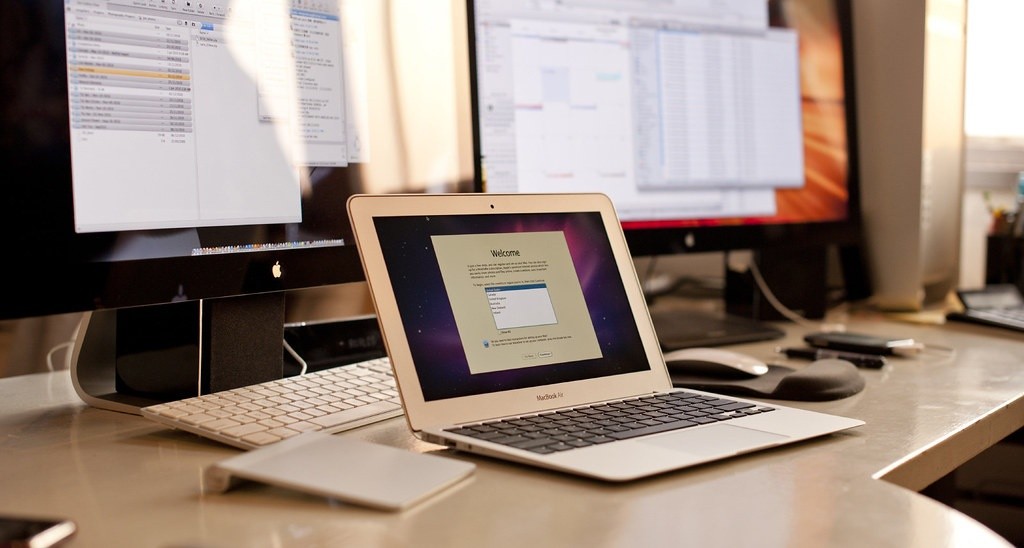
[0,0,855,415]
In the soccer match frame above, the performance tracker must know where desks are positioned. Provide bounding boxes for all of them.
[0,291,1024,548]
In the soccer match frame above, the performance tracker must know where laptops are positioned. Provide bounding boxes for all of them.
[347,194,868,481]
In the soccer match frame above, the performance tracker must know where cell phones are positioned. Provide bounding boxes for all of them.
[804,332,915,354]
[0,513,76,548]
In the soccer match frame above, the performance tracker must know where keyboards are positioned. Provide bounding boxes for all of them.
[139,358,406,450]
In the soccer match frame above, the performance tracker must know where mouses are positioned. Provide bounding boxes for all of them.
[663,348,769,380]
